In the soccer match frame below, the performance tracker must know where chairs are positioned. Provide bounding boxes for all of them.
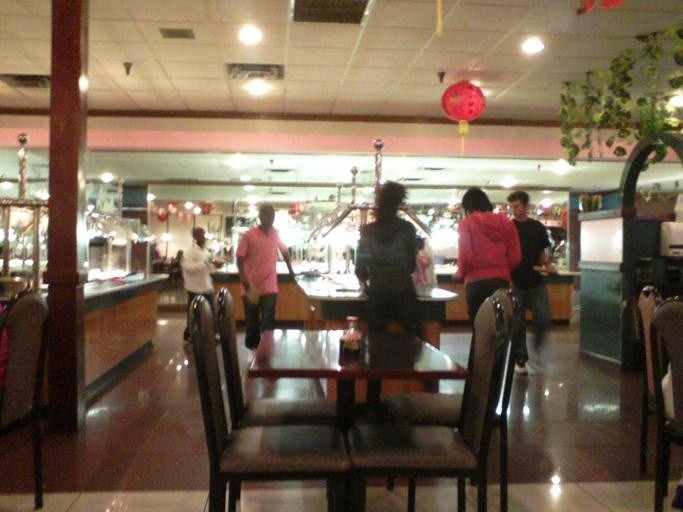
[385,285,522,511]
[637,284,662,470]
[0,292,51,510]
[649,296,683,512]
[188,295,351,512]
[347,295,509,511]
[213,287,338,511]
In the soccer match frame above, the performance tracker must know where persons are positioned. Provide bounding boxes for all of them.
[506,190,560,375]
[171,249,183,283]
[353,178,427,340]
[235,204,295,365]
[180,227,225,344]
[450,186,523,328]
[411,232,430,285]
[153,242,161,261]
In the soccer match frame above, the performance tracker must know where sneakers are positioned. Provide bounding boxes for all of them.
[184,333,192,341]
[514,359,528,374]
[248,347,258,364]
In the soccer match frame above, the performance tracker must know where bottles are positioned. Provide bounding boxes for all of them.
[339,317,363,366]
